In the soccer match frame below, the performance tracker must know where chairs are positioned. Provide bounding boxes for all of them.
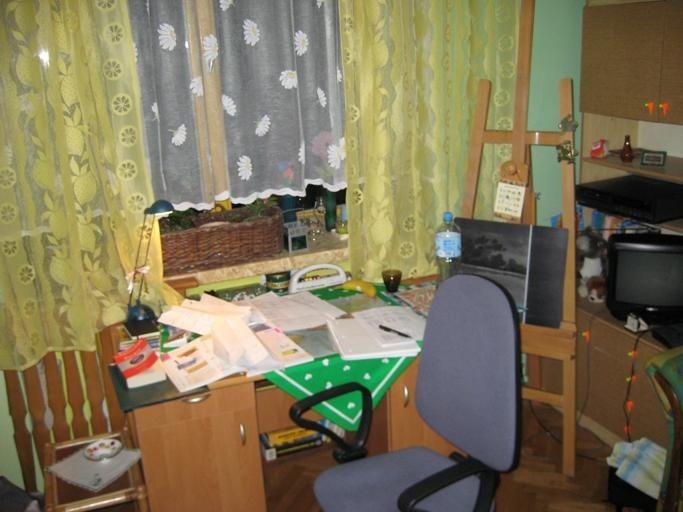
[288,273,523,511]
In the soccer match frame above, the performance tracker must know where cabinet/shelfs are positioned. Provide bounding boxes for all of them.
[124,383,268,511]
[540,2,683,496]
[347,350,466,459]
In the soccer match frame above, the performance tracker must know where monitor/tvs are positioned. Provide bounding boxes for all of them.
[606,233,683,326]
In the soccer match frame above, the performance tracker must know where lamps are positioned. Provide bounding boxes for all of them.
[126,199,174,324]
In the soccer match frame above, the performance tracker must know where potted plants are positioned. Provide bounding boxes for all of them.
[154,196,287,276]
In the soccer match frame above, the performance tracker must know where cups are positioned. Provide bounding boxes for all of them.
[381,270,403,294]
[336,217,348,235]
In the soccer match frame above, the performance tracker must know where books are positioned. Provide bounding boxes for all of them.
[111,282,429,397]
[258,418,349,462]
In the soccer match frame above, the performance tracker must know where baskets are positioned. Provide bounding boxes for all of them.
[158,204,285,276]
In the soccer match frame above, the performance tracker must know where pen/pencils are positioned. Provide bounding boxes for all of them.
[379,323,411,338]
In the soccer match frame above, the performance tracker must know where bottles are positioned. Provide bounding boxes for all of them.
[434,212,463,282]
[620,135,634,164]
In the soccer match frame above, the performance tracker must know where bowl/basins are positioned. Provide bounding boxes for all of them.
[82,438,122,463]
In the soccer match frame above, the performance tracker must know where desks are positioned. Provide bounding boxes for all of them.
[109,274,474,512]
